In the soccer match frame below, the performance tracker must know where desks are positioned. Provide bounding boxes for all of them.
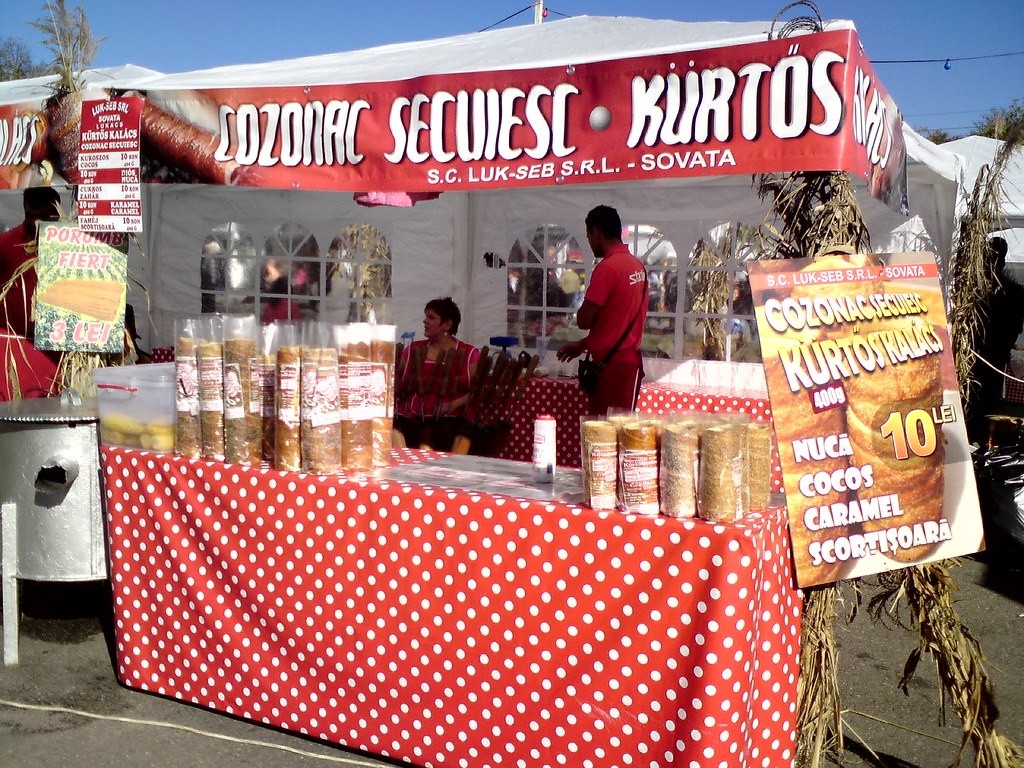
[150,347,786,494]
[98,445,805,768]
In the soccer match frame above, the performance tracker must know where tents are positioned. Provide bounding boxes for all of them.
[0,14,1024,368]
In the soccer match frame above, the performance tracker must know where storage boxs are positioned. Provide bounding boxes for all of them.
[92,361,176,453]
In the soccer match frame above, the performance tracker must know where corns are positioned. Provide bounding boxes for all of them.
[41,281,122,319]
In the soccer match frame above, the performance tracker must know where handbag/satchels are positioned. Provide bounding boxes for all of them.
[576,358,601,392]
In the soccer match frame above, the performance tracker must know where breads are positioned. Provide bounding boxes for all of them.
[175,338,392,476]
[760,322,945,586]
[579,416,771,520]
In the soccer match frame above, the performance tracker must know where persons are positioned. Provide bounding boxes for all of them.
[951,237,1024,469]
[394,296,480,454]
[0,187,61,369]
[556,204,649,417]
